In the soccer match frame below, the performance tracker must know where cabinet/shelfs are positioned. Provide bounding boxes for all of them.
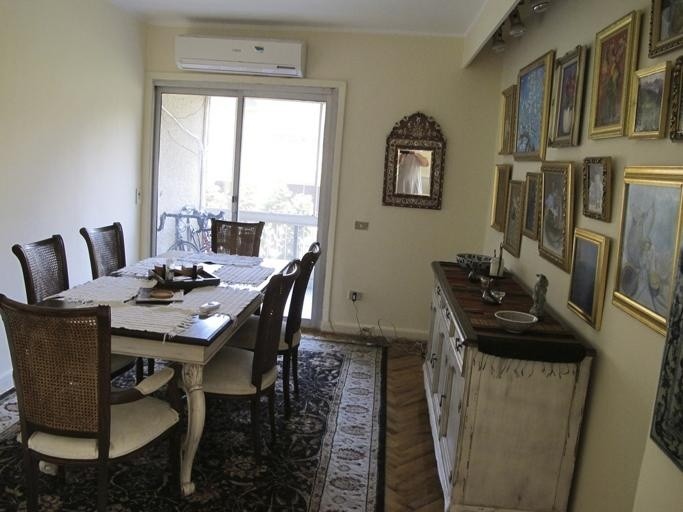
[422,262,593,511]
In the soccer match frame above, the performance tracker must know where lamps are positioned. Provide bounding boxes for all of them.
[531,0,551,13]
[491,28,508,55]
[508,6,528,38]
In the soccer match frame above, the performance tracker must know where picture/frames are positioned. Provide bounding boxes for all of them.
[587,11,641,141]
[627,60,672,138]
[519,173,544,241]
[537,163,575,274]
[546,44,586,149]
[566,226,611,331]
[669,54,683,141]
[611,166,683,337]
[581,157,612,222]
[501,179,529,259]
[648,1,683,59]
[512,50,554,162]
[490,164,512,233]
[496,85,517,154]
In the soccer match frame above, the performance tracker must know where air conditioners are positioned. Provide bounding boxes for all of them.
[172,34,306,78]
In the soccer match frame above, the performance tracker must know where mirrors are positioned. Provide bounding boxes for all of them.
[381,113,446,209]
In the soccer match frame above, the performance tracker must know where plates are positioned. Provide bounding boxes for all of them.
[135,286,185,302]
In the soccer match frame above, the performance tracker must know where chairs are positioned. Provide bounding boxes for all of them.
[211,216,263,259]
[165,258,304,464]
[79,221,126,281]
[223,241,322,413]
[11,234,155,388]
[0,293,181,512]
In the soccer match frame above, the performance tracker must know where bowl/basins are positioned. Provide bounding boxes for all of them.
[494,310,538,332]
[456,252,491,271]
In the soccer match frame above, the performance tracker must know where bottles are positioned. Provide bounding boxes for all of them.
[165,247,177,281]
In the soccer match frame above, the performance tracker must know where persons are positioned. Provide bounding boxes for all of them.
[397,150,429,194]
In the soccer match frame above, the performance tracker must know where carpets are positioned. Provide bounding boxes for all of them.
[0,332,388,512]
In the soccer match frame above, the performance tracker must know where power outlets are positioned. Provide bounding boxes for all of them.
[348,290,361,301]
